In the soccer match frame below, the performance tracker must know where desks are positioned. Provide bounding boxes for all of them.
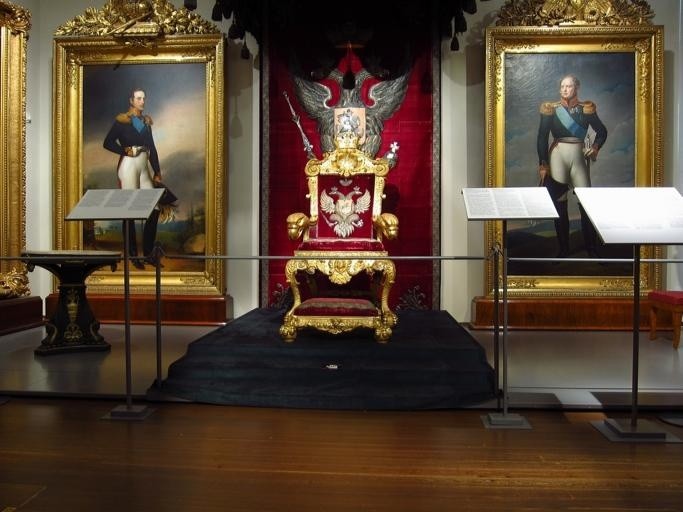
[21,245,125,357]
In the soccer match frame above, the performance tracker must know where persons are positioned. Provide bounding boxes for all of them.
[103,86,164,270]
[536,75,608,258]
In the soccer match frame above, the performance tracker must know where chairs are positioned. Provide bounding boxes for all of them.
[277,128,398,345]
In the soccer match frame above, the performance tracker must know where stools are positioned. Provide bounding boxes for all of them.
[645,286,682,350]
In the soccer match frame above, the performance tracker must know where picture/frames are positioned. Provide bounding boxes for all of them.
[54,0,226,300]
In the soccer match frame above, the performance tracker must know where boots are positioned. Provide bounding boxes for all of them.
[578,201,607,266]
[142,210,166,269]
[552,200,571,267]
[122,219,145,268]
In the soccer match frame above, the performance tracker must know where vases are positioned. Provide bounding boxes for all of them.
[480,0,667,294]
